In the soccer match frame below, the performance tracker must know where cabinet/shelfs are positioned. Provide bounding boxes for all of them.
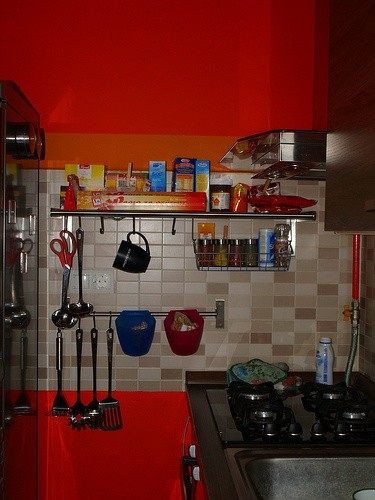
[0,80,47,500]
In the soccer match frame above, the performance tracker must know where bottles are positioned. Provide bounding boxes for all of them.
[198,224,290,268]
[315,338,334,385]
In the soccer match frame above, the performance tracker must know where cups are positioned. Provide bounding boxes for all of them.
[112,232,151,273]
[232,188,247,213]
[114,311,156,356]
[164,309,204,356]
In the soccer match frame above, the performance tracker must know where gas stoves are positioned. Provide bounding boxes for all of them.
[204,382,375,448]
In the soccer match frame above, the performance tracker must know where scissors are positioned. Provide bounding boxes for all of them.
[50,230,76,289]
[6,237,33,289]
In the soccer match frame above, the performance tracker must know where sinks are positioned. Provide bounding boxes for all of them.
[223,445,374,500]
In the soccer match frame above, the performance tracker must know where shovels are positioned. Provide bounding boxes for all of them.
[87,328,99,430]
[13,330,33,417]
[52,327,70,416]
[99,328,122,431]
[67,328,87,431]
